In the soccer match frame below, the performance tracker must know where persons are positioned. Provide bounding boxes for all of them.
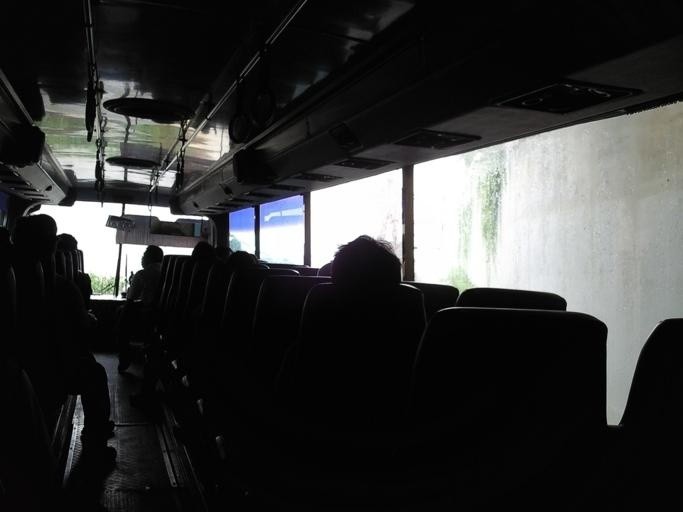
[192,242,214,270]
[127,365,159,410]
[9,217,31,246]
[331,236,403,290]
[216,250,260,294]
[9,214,116,439]
[56,234,93,311]
[111,243,166,372]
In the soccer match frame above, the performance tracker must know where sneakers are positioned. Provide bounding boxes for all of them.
[81,421,115,435]
[118,346,137,371]
[129,392,164,413]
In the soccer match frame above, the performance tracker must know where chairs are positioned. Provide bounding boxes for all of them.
[0,228,681,512]
